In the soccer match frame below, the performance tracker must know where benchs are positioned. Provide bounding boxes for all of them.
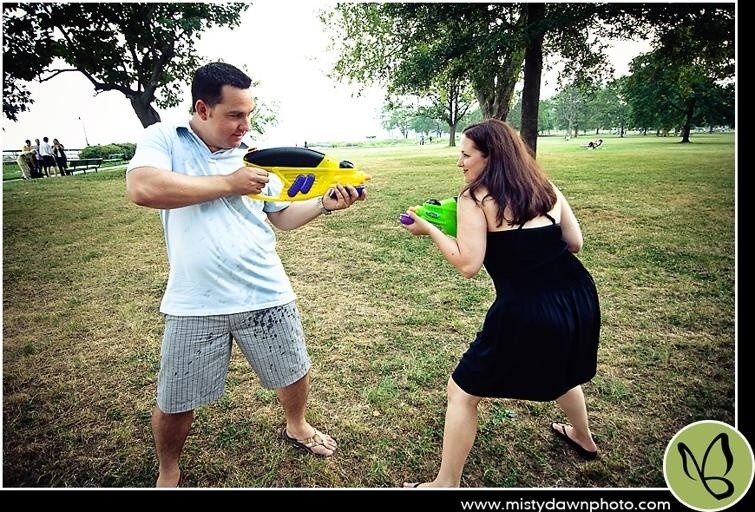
[64,157,103,176]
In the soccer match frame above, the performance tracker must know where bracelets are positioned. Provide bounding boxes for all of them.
[317,197,332,215]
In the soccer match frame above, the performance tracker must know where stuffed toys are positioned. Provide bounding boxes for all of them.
[242,148,366,203]
[398,194,458,240]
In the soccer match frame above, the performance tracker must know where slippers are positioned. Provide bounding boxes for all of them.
[401,475,460,491]
[275,420,336,456]
[548,416,601,460]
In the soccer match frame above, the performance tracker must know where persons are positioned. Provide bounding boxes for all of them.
[401,118,604,489]
[588,139,603,149]
[123,61,372,488]
[15,136,71,180]
